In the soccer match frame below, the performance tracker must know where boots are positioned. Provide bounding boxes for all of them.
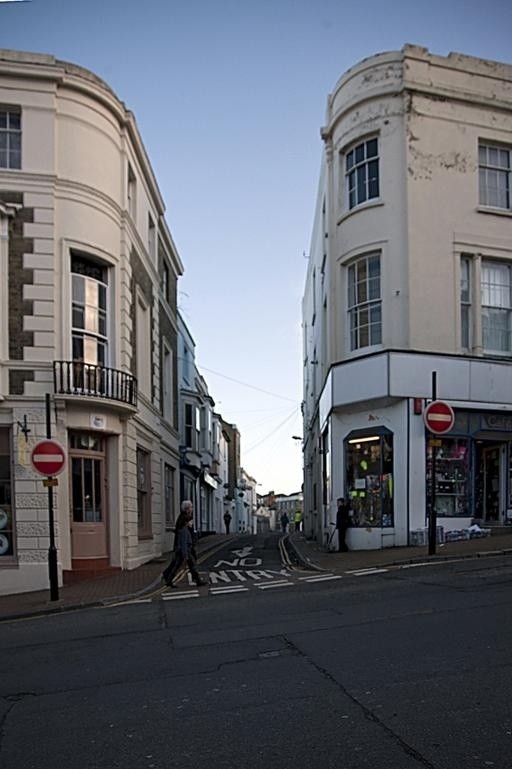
[162,570,211,588]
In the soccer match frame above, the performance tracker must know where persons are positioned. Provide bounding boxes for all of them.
[336,498,353,553]
[294,510,304,532]
[280,512,289,534]
[224,509,232,535]
[161,500,204,582]
[167,513,209,587]
